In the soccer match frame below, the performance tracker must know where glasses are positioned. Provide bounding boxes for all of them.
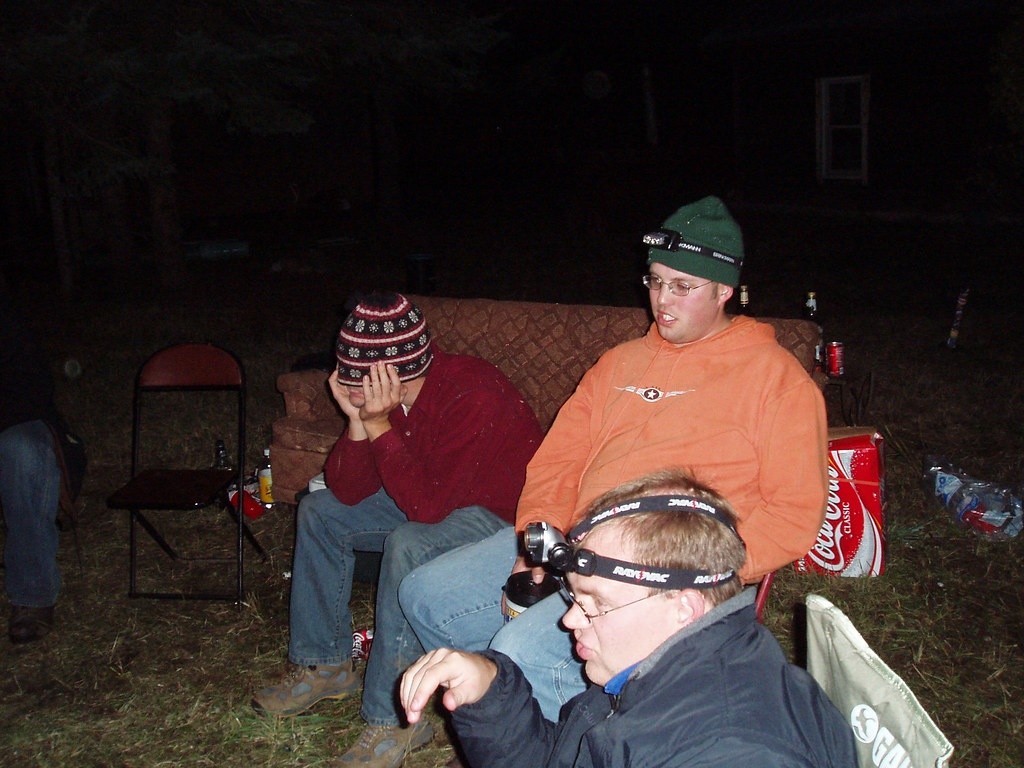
[641,274,715,297]
[556,572,673,624]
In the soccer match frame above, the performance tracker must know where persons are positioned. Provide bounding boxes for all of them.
[0,363,86,643]
[399,198,828,725]
[400,467,859,768]
[252,289,545,768]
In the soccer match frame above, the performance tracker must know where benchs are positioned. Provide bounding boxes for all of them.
[272,295,823,502]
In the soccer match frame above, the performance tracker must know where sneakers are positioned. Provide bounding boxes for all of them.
[331,712,435,768]
[252,659,359,718]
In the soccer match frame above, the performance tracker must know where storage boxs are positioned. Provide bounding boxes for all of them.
[791,426,887,577]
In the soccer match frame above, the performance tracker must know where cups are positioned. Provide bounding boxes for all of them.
[502,571,562,626]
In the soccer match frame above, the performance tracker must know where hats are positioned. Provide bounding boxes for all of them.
[336,286,433,385]
[647,196,745,287]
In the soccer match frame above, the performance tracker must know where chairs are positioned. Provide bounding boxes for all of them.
[105,344,266,613]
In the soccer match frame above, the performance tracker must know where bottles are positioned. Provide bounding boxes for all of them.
[229,489,264,519]
[736,284,750,315]
[258,449,276,503]
[215,439,228,468]
[802,291,819,324]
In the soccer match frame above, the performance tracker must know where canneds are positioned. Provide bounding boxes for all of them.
[825,341,845,377]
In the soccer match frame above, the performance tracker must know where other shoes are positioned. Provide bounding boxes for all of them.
[6,603,54,642]
